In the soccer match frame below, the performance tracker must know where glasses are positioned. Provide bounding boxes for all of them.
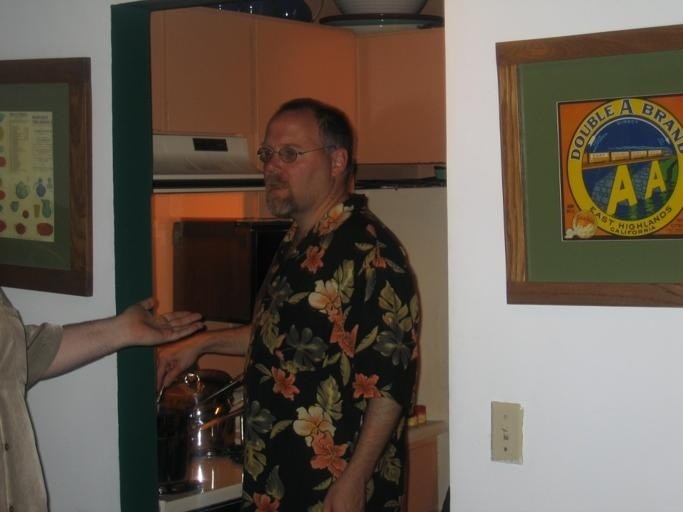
[255,145,339,162]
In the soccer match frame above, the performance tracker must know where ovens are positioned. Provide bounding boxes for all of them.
[149,188,292,377]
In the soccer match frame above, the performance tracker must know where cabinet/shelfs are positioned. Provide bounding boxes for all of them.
[152,6,253,138]
[252,16,356,176]
[356,26,445,165]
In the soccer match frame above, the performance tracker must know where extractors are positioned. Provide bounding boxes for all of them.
[152,134,263,186]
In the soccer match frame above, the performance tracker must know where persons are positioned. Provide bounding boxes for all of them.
[0,282,206,511]
[156,98,421,512]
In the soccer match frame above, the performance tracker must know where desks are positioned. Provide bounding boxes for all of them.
[156,420,448,512]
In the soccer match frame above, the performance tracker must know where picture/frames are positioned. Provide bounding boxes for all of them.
[0,55,93,297]
[494,24,683,307]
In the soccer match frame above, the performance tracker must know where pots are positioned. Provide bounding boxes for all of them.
[155,365,243,498]
